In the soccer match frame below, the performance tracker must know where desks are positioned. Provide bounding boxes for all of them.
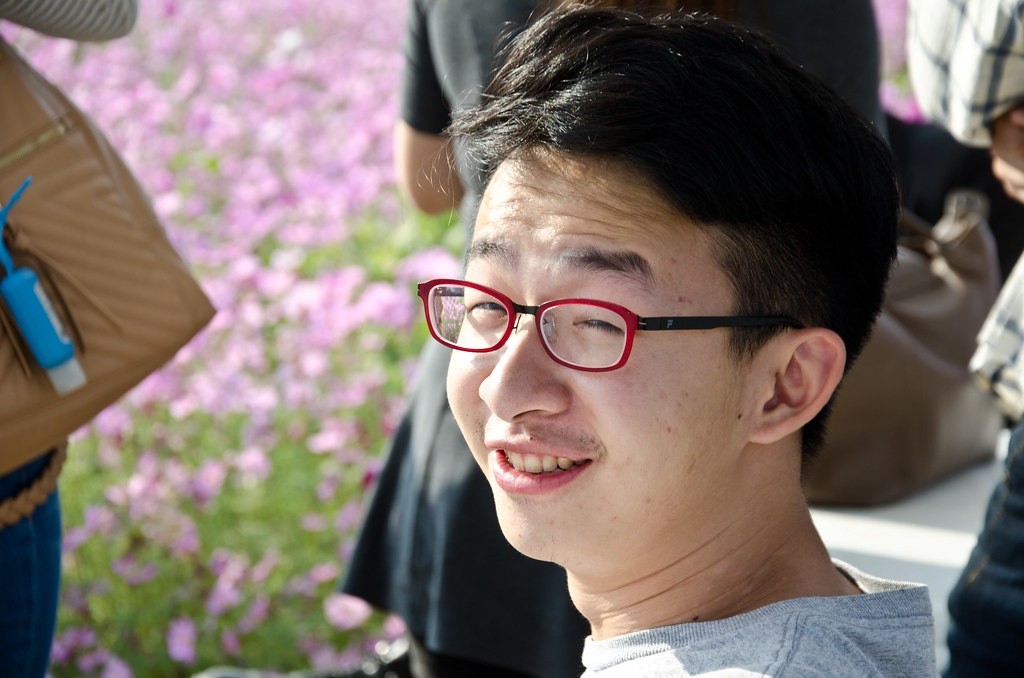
[808,428,1015,673]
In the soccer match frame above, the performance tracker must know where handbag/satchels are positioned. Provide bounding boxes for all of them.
[0,35,218,474]
[800,194,1000,511]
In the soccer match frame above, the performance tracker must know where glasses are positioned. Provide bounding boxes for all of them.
[417,279,807,372]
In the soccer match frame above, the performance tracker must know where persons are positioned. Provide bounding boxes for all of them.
[0,0,138,678]
[447,0,936,678]
[908,0,1024,678]
[341,0,881,678]
